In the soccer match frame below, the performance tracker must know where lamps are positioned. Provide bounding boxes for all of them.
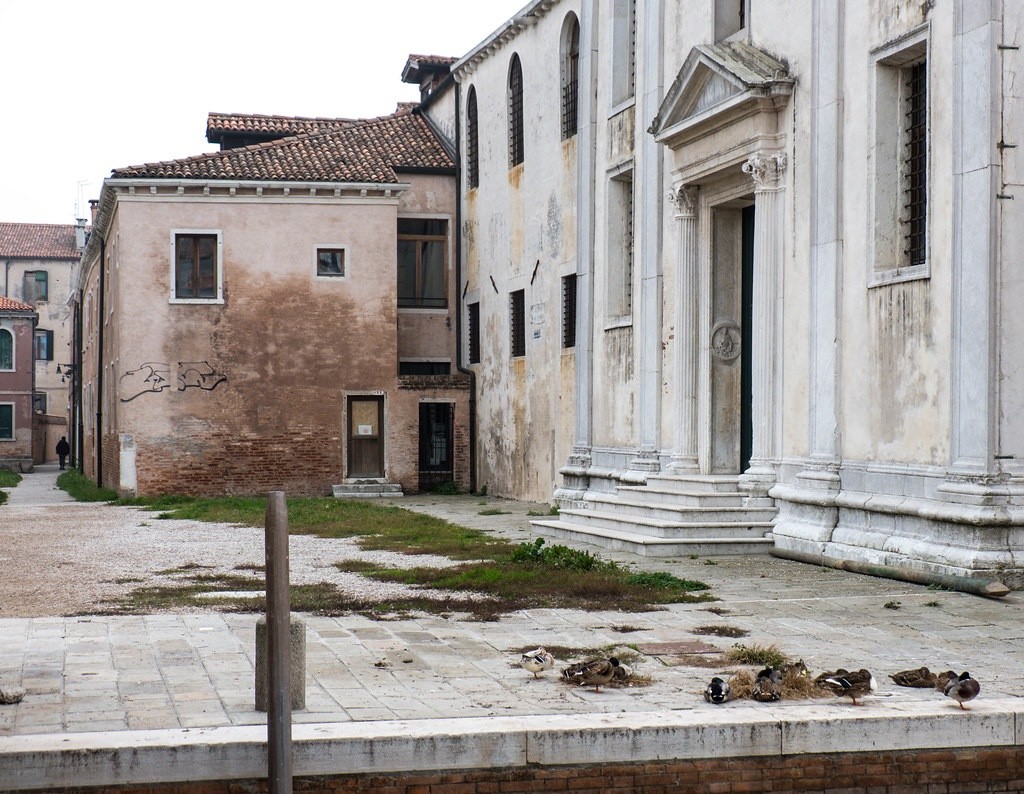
[60,376,70,384]
[55,362,77,374]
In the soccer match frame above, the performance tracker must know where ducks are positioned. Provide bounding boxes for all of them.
[936,670,958,693]
[821,668,878,706]
[814,669,850,690]
[703,677,734,704]
[520,646,555,680]
[750,659,807,701]
[943,672,980,710]
[887,666,937,688]
[560,656,633,694]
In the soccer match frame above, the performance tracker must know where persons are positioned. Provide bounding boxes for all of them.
[56,436,70,470]
